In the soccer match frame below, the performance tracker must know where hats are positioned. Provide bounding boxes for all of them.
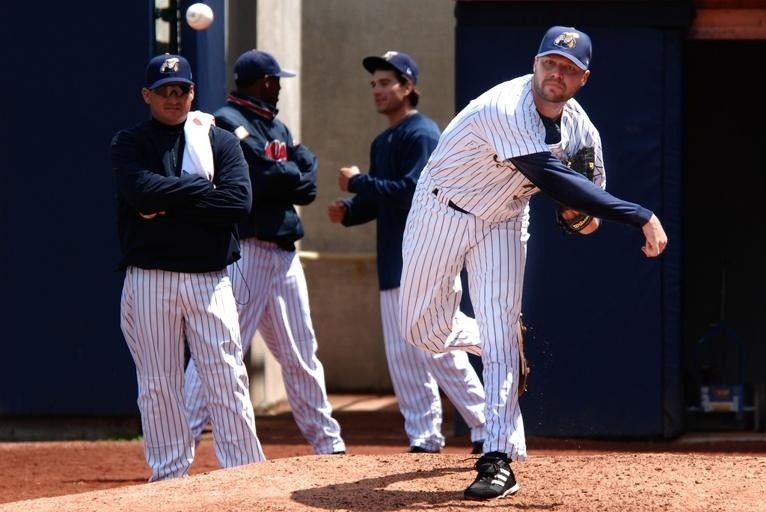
[361,51,419,82]
[233,50,295,81]
[535,26,592,71]
[145,52,196,90]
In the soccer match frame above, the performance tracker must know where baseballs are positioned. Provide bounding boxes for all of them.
[186,3,213,31]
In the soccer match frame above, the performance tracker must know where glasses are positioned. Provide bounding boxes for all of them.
[152,84,193,98]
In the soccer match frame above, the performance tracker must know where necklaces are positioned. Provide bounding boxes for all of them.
[542,103,563,136]
[383,118,406,142]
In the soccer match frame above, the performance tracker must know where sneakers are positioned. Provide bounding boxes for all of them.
[518,314,530,397]
[407,446,441,452]
[470,439,484,454]
[464,451,518,500]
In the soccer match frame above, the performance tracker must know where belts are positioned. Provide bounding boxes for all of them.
[433,188,469,214]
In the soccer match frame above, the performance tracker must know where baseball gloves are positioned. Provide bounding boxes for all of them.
[555,147,595,234]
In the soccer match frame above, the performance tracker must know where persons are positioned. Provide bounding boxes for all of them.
[325,48,487,456]
[107,50,268,483]
[181,48,348,459]
[396,24,669,503]
